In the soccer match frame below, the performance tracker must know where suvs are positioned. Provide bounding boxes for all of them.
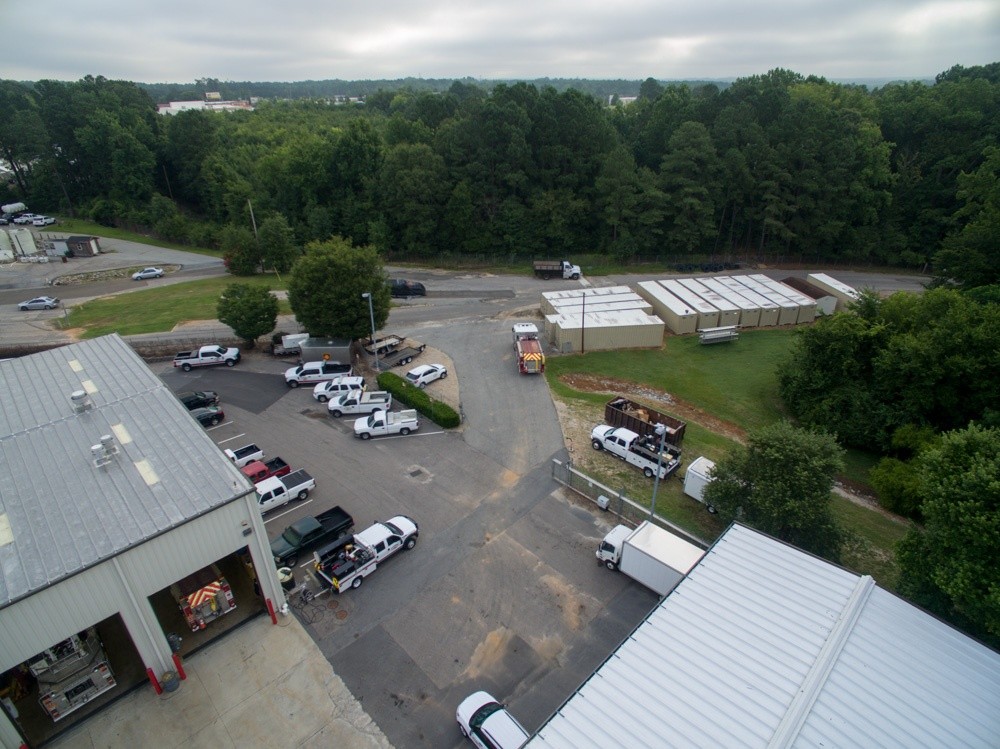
[176,389,221,411]
[313,376,368,403]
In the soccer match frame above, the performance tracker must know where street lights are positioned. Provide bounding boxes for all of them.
[648,423,668,522]
[362,293,380,375]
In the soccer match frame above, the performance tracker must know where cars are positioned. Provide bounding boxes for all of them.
[17,296,61,311]
[132,266,165,281]
[0,210,57,227]
[385,278,426,299]
[188,406,225,427]
[454,689,530,749]
[404,363,447,390]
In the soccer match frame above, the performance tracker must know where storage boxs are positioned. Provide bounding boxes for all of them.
[540,273,882,354]
[605,395,687,446]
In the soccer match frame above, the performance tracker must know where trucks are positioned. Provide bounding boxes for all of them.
[596,519,707,599]
[533,260,582,280]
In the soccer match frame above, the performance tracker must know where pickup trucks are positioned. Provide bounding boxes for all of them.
[353,409,420,440]
[327,388,392,419]
[254,467,318,516]
[283,359,354,388]
[590,422,684,482]
[172,343,241,372]
[240,456,292,486]
[312,513,420,595]
[269,506,356,568]
[223,442,266,470]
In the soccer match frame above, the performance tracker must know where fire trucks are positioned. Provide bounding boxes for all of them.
[26,625,118,723]
[511,322,546,375]
[168,563,238,633]
